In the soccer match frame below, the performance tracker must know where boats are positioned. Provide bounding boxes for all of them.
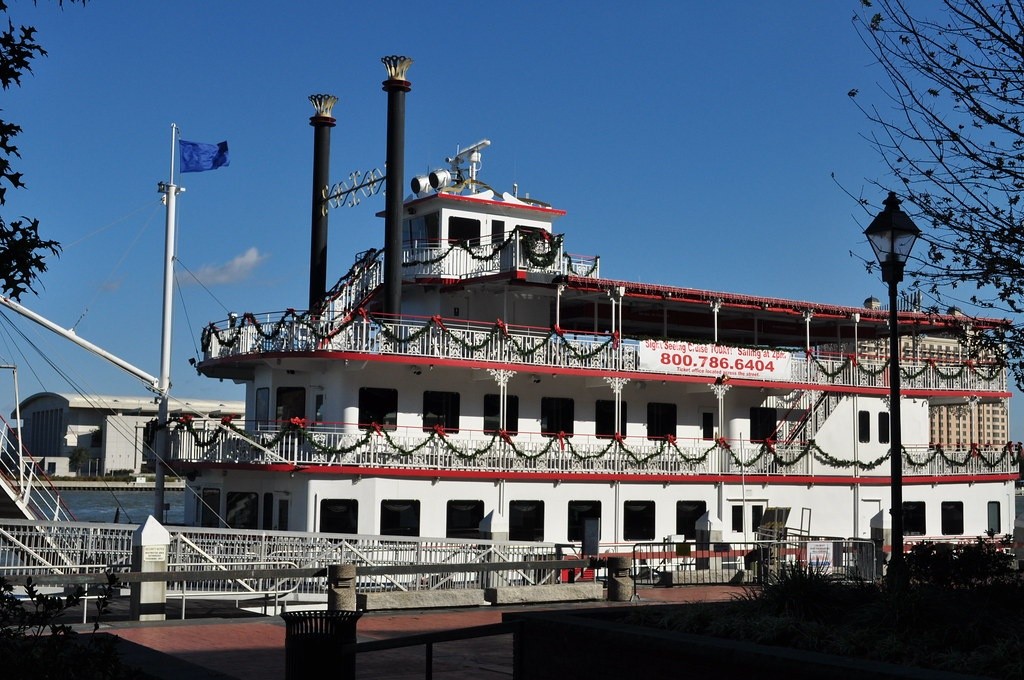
[147,50,1015,620]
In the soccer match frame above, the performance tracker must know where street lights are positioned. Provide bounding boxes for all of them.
[864,192,924,607]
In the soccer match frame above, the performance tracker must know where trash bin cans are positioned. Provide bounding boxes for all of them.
[279,609,364,680]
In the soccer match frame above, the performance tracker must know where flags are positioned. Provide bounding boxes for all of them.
[179,139,230,173]
